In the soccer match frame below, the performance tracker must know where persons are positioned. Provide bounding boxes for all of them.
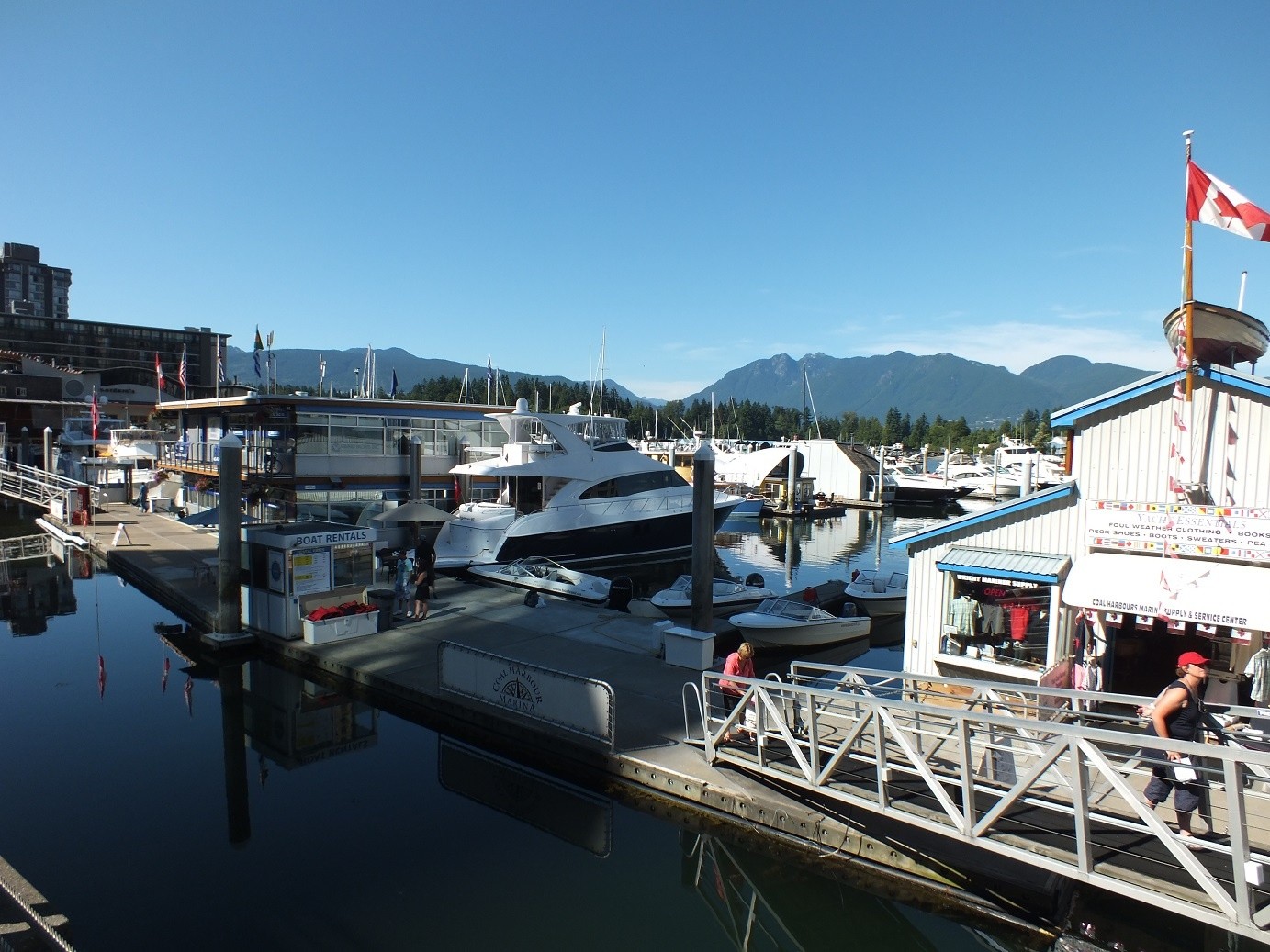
[1136,651,1212,851]
[391,540,438,620]
[719,642,756,742]
[137,482,149,512]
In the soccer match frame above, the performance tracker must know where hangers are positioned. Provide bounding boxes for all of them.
[1263,641,1269,649]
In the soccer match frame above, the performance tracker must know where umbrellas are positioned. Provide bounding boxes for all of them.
[367,499,462,577]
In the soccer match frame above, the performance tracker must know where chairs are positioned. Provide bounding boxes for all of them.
[544,570,558,580]
[874,578,886,594]
[190,556,213,587]
[380,546,415,583]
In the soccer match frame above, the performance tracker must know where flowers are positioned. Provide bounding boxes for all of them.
[194,477,213,493]
[153,470,168,484]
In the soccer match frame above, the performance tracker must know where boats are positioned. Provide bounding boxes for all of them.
[687,471,772,517]
[727,597,871,649]
[465,555,634,611]
[648,573,775,618]
[869,437,1065,507]
[531,433,741,482]
[843,569,908,617]
[419,412,748,570]
[51,412,181,501]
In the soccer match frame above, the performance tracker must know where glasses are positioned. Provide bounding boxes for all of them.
[743,656,750,660]
[1188,663,1205,668]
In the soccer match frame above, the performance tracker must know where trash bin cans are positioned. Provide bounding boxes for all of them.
[367,589,396,630]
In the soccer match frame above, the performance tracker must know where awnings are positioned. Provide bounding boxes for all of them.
[1061,552,1270,631]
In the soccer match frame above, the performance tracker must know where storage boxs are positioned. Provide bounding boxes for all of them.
[300,610,380,645]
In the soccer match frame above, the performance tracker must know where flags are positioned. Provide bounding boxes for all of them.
[91,391,100,438]
[155,353,166,390]
[1185,160,1270,243]
[390,370,398,397]
[218,336,225,382]
[99,654,193,712]
[178,348,187,388]
[487,359,493,387]
[253,329,264,378]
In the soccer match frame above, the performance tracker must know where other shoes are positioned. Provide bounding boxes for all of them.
[392,610,403,616]
[406,611,412,618]
[432,592,438,599]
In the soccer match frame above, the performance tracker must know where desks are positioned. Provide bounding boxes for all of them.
[383,556,399,577]
[202,557,218,581]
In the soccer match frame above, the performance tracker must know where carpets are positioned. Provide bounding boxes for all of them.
[1061,717,1145,764]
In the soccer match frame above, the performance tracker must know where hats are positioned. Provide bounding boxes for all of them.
[1177,651,1211,668]
